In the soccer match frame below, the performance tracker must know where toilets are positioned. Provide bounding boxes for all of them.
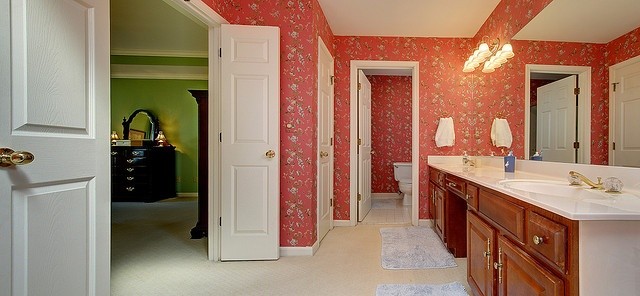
[393,162,412,206]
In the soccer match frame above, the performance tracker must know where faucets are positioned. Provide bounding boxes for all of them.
[465,160,475,167]
[569,170,604,190]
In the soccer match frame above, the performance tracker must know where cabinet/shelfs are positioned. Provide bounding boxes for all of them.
[467,187,579,296]
[428,167,467,258]
[111,145,177,203]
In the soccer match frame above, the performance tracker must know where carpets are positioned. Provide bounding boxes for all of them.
[372,198,395,210]
[379,226,457,269]
[375,281,470,296]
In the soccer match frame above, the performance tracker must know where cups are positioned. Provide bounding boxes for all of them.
[531,156,542,161]
[504,156,515,173]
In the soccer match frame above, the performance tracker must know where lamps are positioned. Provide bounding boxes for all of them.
[155,131,165,146]
[482,42,514,73]
[110,131,119,144]
[462,35,500,73]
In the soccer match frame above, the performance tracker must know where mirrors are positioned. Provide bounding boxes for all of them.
[473,0,640,168]
[120,109,159,142]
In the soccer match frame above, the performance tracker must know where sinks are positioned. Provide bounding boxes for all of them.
[442,165,472,172]
[499,179,611,199]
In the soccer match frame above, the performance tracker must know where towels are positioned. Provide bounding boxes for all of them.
[434,117,456,148]
[490,118,513,149]
